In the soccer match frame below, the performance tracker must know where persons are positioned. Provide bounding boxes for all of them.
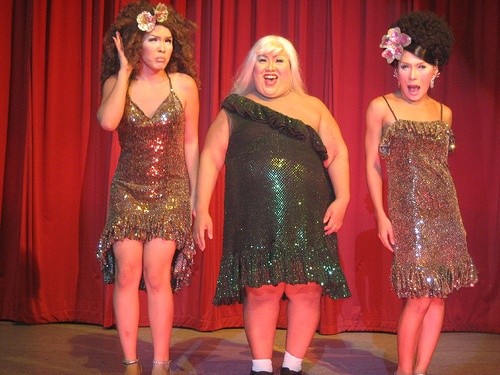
[196,35,351,375]
[363,11,480,375]
[97,2,200,375]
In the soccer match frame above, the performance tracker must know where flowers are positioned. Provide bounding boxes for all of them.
[151,2,169,23]
[379,27,411,64]
[136,11,156,32]
